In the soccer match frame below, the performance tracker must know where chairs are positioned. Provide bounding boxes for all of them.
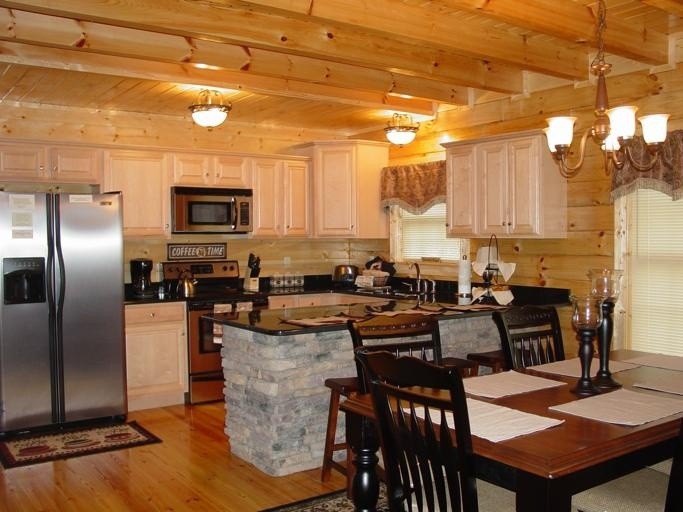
[491,305,565,371]
[346,312,456,495]
[353,345,482,512]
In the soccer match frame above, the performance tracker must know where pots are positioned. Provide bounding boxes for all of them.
[331,264,358,286]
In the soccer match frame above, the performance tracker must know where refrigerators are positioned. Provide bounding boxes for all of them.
[2,189,126,435]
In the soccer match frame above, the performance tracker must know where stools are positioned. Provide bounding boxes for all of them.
[320,376,360,500]
[467,348,533,375]
[428,357,480,377]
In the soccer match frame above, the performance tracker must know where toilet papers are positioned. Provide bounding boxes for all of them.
[459,260,472,295]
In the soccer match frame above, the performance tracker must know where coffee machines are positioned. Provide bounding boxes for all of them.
[129,257,156,298]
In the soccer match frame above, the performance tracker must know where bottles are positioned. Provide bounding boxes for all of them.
[158,294,164,299]
[457,255,472,298]
[158,285,166,294]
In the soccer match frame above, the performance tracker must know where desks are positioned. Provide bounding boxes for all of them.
[338,348,683,512]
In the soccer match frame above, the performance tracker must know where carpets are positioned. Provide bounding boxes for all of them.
[257,457,415,512]
[0,418,164,470]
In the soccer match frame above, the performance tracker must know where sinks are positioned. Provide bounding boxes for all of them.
[395,291,426,299]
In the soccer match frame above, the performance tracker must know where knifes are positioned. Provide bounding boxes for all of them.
[248,253,262,278]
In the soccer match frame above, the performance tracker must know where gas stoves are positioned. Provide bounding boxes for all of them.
[174,284,258,302]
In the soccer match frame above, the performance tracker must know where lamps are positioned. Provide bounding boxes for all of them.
[186,88,232,132]
[540,0,674,179]
[384,110,421,145]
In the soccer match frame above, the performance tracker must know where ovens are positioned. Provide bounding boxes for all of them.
[188,305,267,375]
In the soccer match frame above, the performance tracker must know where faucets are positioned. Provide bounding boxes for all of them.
[408,262,421,291]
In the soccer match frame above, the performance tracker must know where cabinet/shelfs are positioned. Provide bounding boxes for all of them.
[479,128,541,239]
[440,141,480,240]
[126,300,189,412]
[1,139,102,184]
[253,154,309,239]
[336,293,383,305]
[315,138,388,239]
[168,149,253,188]
[268,293,335,310]
[101,145,170,237]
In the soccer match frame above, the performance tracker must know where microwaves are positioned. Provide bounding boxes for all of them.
[170,188,252,236]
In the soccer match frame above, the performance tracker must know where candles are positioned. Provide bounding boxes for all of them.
[596,277,613,297]
[575,304,599,327]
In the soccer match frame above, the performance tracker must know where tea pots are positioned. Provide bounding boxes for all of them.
[172,270,201,296]
